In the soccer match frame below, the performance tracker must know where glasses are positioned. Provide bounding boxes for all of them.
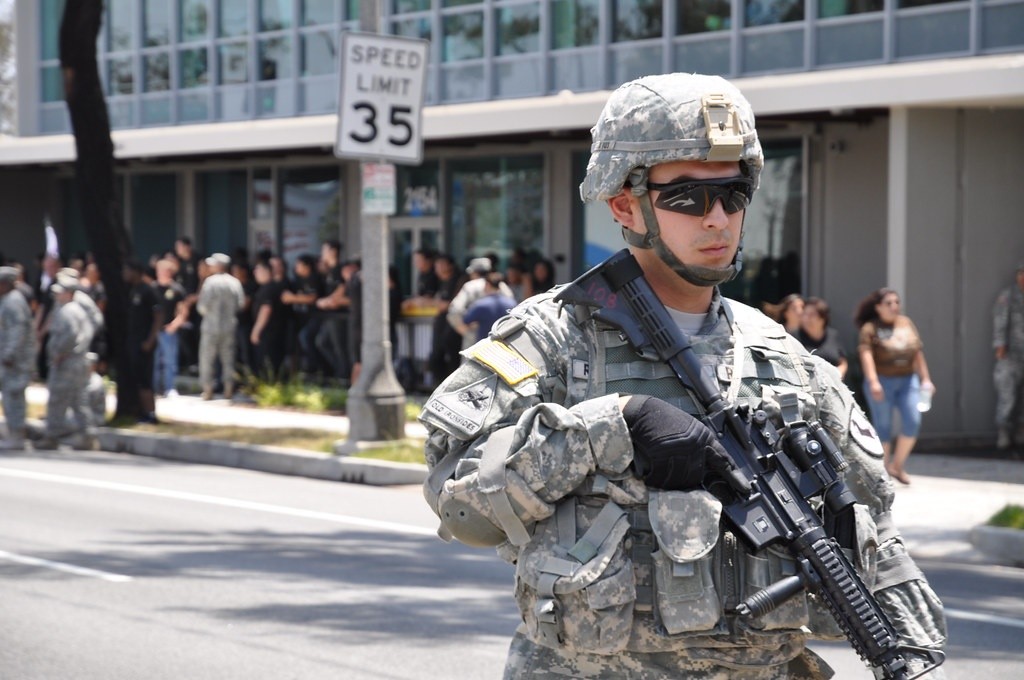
[645,174,755,216]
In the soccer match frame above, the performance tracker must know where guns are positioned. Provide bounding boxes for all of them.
[552,247,947,680]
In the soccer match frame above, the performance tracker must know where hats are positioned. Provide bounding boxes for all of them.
[50,268,81,294]
[466,258,491,274]
[86,352,98,365]
[205,253,230,265]
[0,267,17,280]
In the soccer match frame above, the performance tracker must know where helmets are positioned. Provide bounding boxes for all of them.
[579,72,765,204]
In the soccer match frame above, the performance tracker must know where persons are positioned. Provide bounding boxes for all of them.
[763,294,804,341]
[0,236,556,449]
[857,289,936,484]
[791,296,848,381]
[418,73,948,680]
[991,266,1024,450]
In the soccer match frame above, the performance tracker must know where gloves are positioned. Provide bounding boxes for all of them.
[622,393,752,500]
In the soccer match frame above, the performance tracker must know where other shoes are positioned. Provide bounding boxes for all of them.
[73,438,100,451]
[0,434,24,449]
[887,465,912,485]
[997,429,1010,447]
[163,388,178,398]
[201,391,213,400]
[33,436,59,450]
[223,390,232,398]
